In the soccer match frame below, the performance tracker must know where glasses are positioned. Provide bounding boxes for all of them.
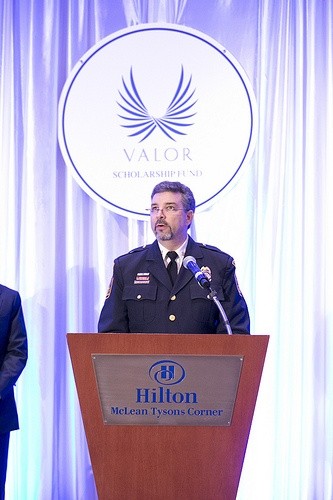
[146,207,189,215]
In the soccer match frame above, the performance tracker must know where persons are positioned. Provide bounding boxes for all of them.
[97,180,250,335]
[0,284,28,500]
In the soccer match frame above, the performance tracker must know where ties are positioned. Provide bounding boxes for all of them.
[166,251,178,287]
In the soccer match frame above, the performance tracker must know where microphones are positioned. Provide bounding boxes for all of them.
[182,256,210,289]
